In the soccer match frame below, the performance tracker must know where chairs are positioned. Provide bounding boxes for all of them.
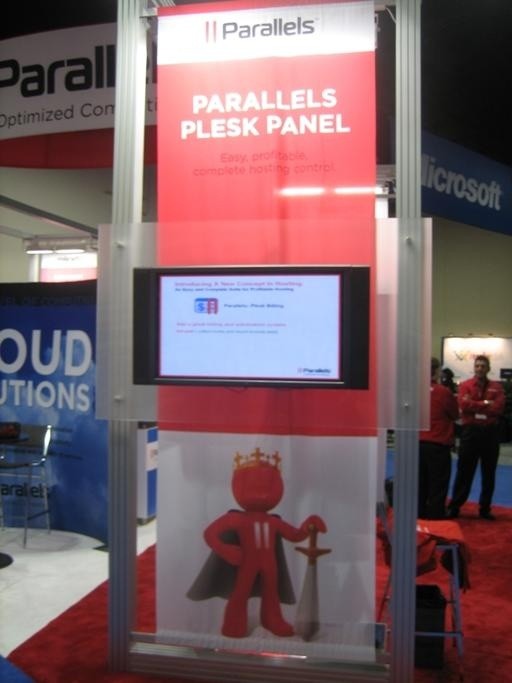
[0,424,53,547]
[376,480,472,657]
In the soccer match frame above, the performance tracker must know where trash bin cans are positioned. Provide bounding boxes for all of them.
[414,584,447,670]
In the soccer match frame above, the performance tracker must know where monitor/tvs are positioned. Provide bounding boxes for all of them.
[134,267,369,389]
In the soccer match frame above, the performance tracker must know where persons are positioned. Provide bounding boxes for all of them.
[445,351,506,522]
[438,366,457,393]
[414,355,461,522]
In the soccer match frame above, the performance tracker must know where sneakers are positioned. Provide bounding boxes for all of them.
[444,508,496,518]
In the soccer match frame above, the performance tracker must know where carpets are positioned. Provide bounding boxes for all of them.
[5,495,511,682]
